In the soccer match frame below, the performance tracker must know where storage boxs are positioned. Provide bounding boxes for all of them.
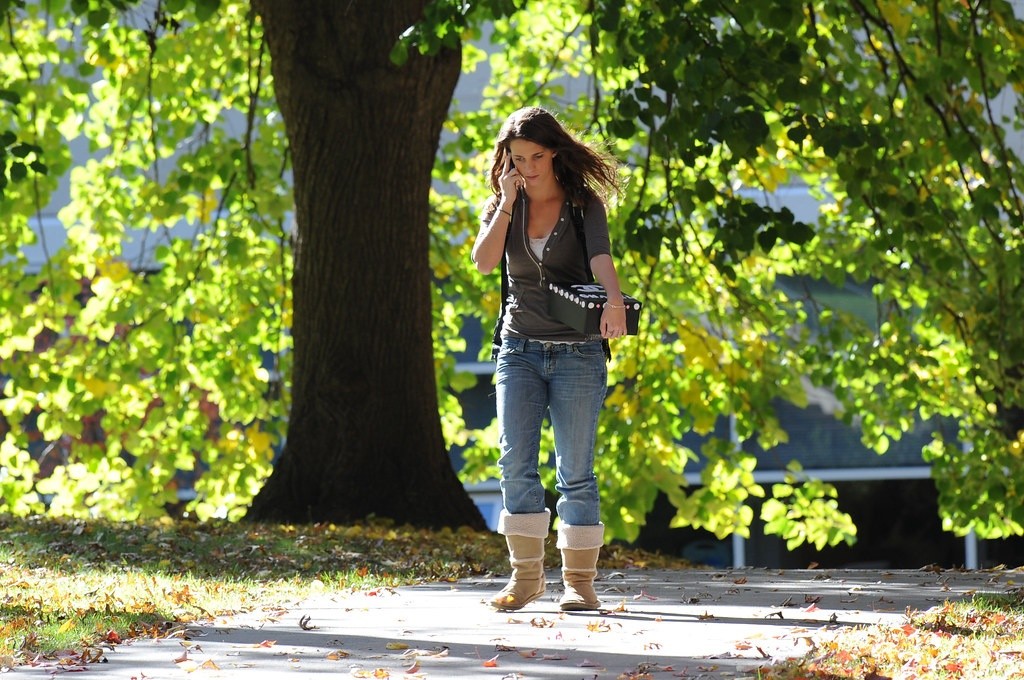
[550,282,642,336]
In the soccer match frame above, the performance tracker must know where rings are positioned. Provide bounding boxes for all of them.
[606,331,613,336]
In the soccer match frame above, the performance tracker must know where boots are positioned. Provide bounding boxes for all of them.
[555,522,604,610]
[490,507,550,610]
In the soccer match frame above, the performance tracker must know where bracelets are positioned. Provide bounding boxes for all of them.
[606,302,626,309]
[497,207,511,216]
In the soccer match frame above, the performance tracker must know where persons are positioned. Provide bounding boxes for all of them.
[472,106,629,610]
